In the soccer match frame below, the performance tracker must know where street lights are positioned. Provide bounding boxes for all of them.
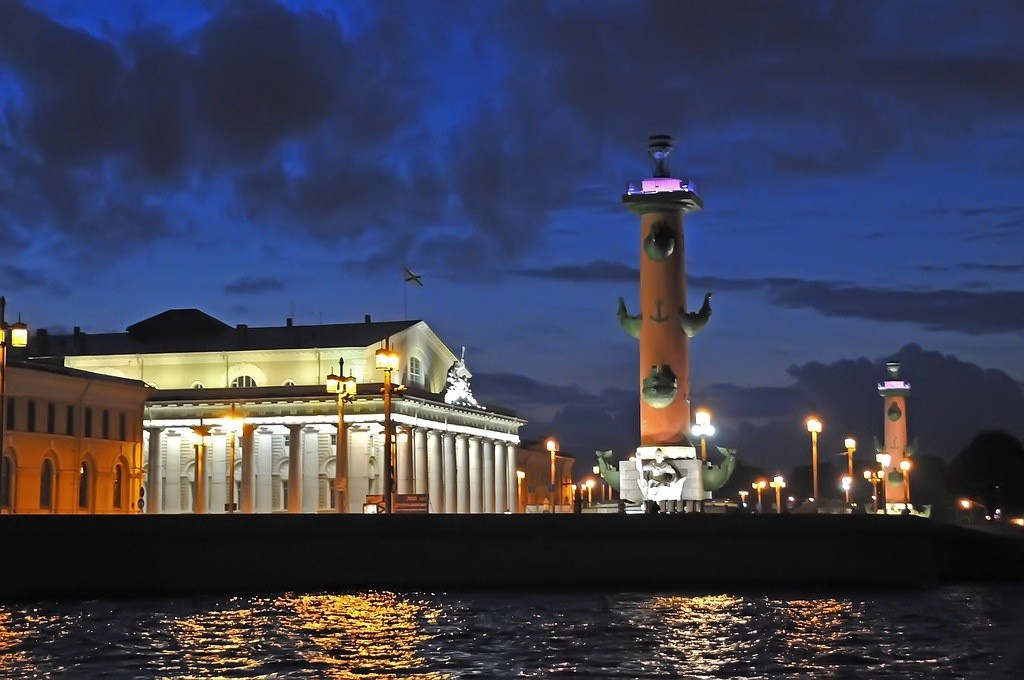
[221,403,245,512]
[843,438,856,501]
[739,490,749,504]
[326,357,357,512]
[864,470,884,514]
[375,332,400,514]
[900,460,910,505]
[516,470,526,512]
[751,481,767,511]
[546,441,560,513]
[585,480,595,508]
[876,453,892,515]
[841,475,852,502]
[807,419,823,499]
[0,296,28,514]
[770,477,787,514]
[691,411,715,463]
